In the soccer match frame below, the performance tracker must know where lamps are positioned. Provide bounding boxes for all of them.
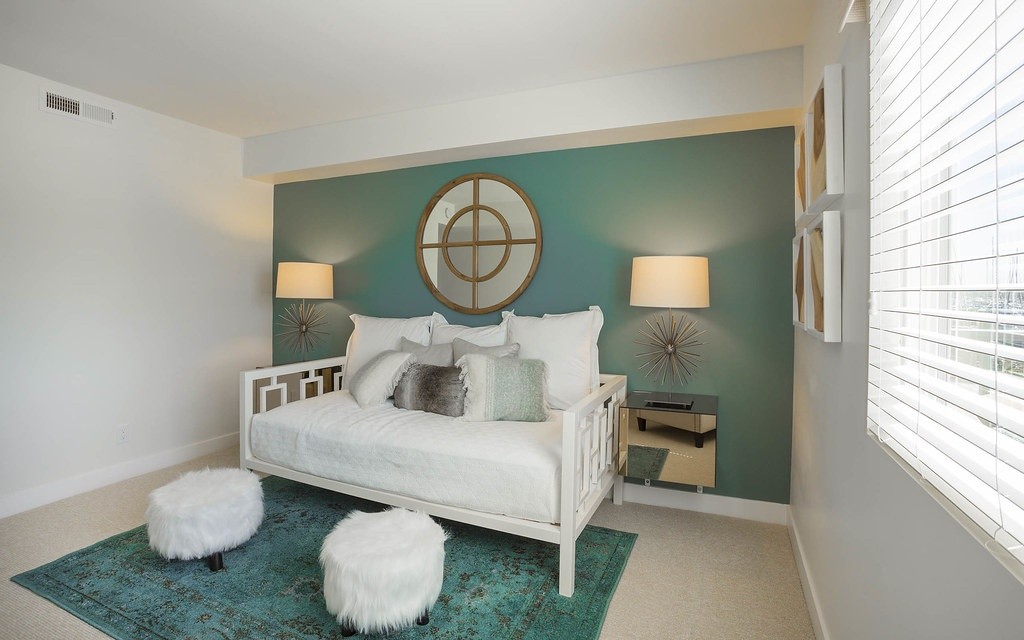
[631,256,710,354]
[275,262,333,334]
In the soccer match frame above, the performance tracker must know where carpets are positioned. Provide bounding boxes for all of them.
[9,474,638,640]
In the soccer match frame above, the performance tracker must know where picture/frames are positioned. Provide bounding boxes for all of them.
[790,64,843,344]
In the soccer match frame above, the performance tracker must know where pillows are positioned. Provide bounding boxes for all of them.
[394,360,465,416]
[341,313,433,391]
[449,338,520,369]
[499,305,604,410]
[399,337,453,366]
[452,353,550,423]
[349,349,417,408]
[429,308,516,346]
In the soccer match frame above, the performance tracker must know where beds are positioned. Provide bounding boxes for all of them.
[239,353,627,597]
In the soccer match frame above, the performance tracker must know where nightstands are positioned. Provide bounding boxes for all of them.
[302,364,342,397]
[616,390,719,490]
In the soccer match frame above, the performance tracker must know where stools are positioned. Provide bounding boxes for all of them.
[146,467,265,571]
[315,504,452,634]
